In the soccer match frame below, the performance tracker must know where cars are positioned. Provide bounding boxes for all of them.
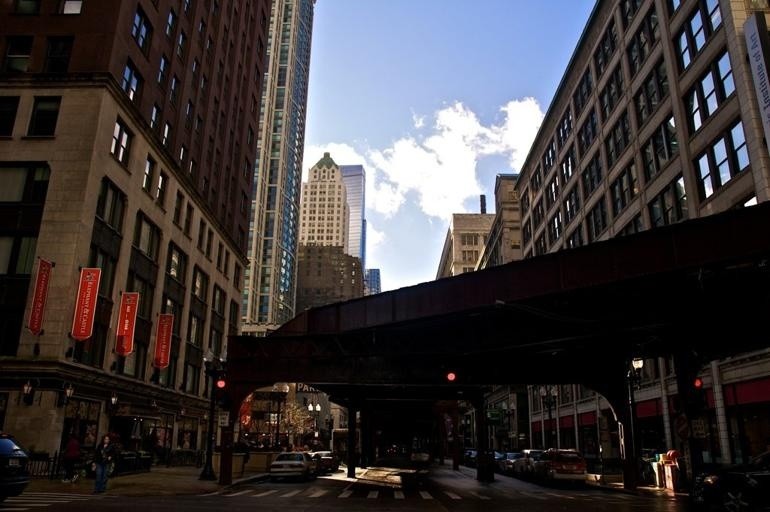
[270,450,340,483]
[0,431,32,504]
[462,449,588,488]
[700,451,770,512]
[411,448,432,463]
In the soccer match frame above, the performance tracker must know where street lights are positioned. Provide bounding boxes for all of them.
[539,385,559,448]
[308,402,321,450]
[325,414,334,451]
[198,343,229,481]
[461,419,471,449]
[627,357,645,480]
[271,383,289,449]
[502,401,517,450]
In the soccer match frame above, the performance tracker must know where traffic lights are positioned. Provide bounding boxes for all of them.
[431,369,456,383]
[216,371,227,407]
[692,376,705,416]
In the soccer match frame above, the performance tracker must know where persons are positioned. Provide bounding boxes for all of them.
[89,433,116,496]
[57,429,79,484]
